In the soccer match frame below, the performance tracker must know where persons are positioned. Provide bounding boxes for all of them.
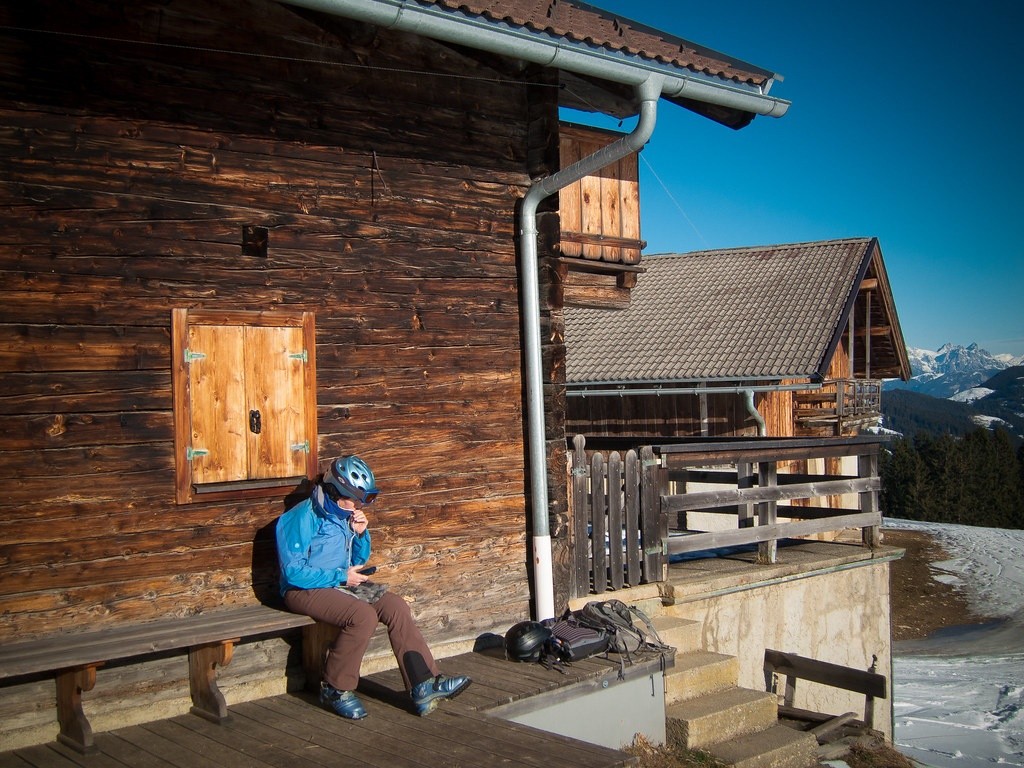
[276,456,472,717]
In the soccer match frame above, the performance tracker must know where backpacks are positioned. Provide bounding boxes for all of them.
[582,599,667,654]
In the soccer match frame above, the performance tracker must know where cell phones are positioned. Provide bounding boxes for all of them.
[357,567,376,576]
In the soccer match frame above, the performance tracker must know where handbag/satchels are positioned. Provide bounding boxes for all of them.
[542,612,613,663]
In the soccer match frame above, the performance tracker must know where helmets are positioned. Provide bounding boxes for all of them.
[503,620,552,657]
[321,455,376,503]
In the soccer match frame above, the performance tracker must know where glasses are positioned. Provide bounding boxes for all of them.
[362,491,379,503]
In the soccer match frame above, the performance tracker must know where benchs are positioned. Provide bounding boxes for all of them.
[0,601,345,755]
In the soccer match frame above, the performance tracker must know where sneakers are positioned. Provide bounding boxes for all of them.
[320,680,368,720]
[410,674,472,718]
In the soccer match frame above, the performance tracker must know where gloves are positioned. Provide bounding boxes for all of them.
[348,579,389,603]
